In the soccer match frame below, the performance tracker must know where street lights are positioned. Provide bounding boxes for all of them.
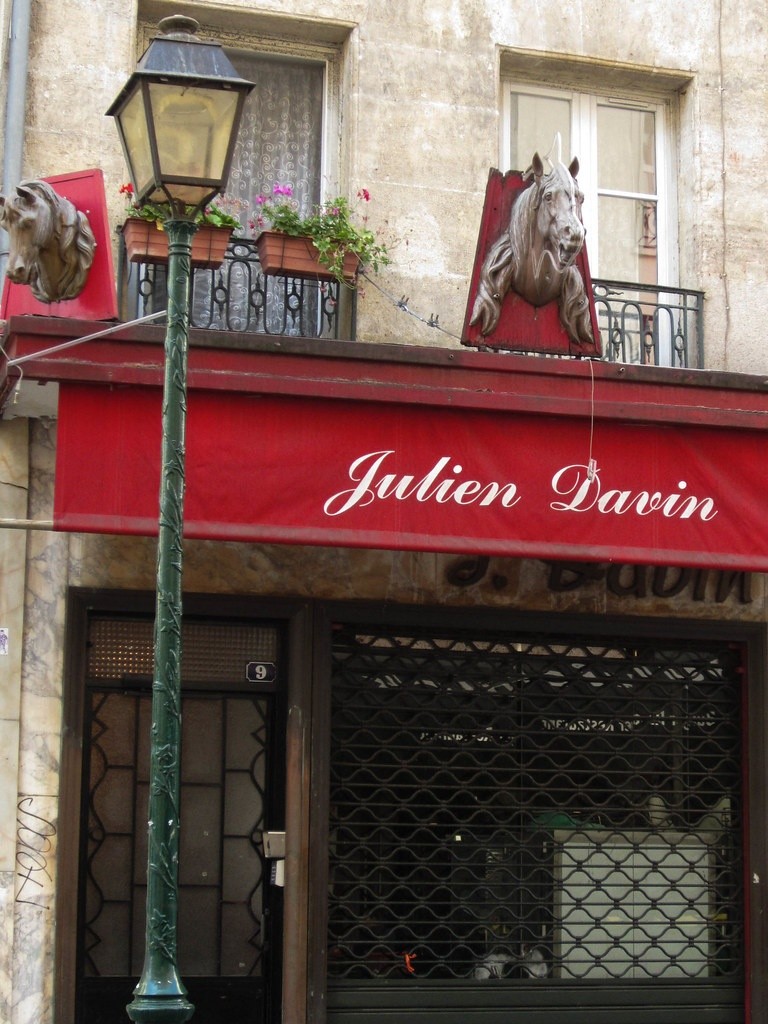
[102,12,260,1024]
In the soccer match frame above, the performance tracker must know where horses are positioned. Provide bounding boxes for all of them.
[469,156,598,346]
[0,178,96,303]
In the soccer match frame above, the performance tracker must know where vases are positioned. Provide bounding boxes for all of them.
[254,232,362,283]
[121,218,236,269]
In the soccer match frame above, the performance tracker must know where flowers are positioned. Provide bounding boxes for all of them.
[247,180,417,301]
[117,183,248,236]
